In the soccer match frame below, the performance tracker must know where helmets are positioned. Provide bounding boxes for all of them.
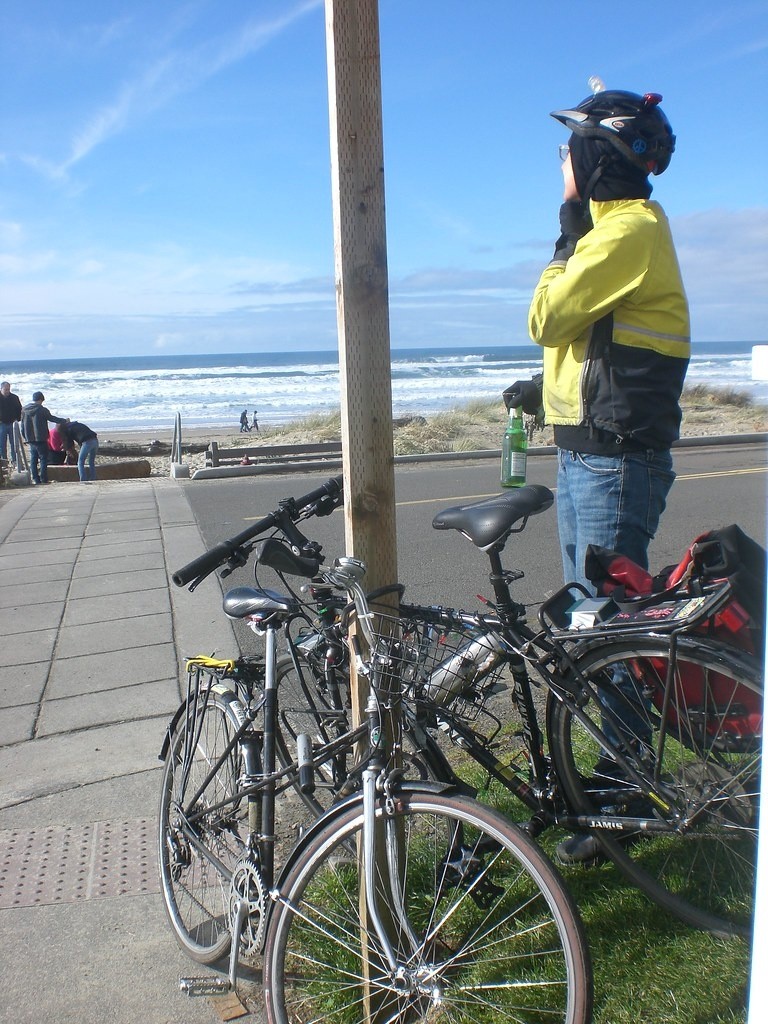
[549,75,676,176]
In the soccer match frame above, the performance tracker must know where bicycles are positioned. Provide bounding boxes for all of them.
[154,472,765,1024]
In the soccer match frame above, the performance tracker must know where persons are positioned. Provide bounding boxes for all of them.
[0,381,22,469]
[239,410,259,433]
[502,88,692,868]
[21,391,99,485]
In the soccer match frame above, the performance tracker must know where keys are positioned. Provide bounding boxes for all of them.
[522,410,539,440]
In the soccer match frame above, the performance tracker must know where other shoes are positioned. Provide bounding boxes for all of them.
[555,803,669,867]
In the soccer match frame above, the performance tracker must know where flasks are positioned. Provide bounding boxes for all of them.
[421,628,507,707]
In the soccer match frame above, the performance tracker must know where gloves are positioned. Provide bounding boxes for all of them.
[559,200,590,240]
[503,381,542,415]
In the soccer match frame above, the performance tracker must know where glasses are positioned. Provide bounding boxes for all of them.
[559,145,570,160]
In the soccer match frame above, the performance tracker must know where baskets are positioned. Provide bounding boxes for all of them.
[370,612,507,721]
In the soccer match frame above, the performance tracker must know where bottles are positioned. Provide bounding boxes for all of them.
[500,392,527,489]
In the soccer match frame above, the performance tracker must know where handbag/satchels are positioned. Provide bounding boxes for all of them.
[586,524,768,753]
